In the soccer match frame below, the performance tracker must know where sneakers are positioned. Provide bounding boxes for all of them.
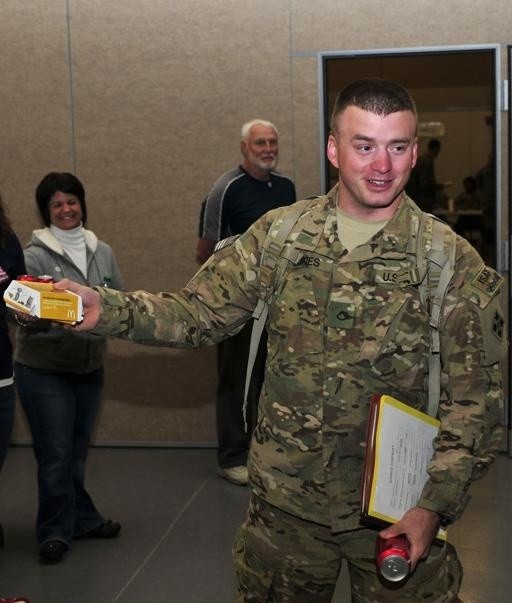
[39,540,64,560]
[216,465,248,485]
[78,519,121,538]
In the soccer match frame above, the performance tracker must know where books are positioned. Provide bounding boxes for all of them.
[356,390,449,547]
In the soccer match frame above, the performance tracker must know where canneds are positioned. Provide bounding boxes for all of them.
[38,274,54,284]
[375,534,411,582]
[17,275,37,282]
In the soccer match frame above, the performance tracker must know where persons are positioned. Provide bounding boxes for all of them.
[191,118,300,490]
[406,136,451,221]
[453,174,480,210]
[49,76,512,603]
[15,169,124,570]
[1,193,27,547]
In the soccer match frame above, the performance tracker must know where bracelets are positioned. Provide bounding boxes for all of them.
[14,311,33,326]
